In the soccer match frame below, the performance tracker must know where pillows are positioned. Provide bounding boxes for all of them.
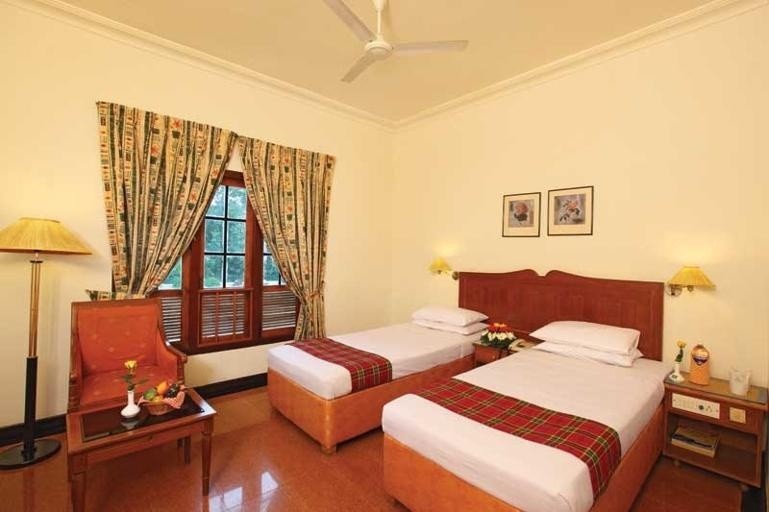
[531,342,643,367]
[412,307,489,326]
[414,320,489,335]
[528,321,641,356]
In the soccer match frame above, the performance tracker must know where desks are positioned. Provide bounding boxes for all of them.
[66,389,217,512]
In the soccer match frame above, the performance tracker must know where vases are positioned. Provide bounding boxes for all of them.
[120,389,140,419]
[491,342,508,348]
[668,362,684,384]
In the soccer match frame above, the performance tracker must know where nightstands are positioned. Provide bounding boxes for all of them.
[662,369,769,493]
[472,340,514,367]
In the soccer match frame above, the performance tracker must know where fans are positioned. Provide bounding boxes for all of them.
[324,0,471,85]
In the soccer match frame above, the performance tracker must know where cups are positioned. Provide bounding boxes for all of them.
[729,368,751,397]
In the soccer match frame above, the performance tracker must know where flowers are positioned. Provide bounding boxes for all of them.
[674,339,687,362]
[115,361,148,390]
[480,323,517,344]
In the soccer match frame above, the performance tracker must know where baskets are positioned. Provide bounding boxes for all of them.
[141,402,183,415]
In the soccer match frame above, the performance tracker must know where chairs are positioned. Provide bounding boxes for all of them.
[68,297,189,448]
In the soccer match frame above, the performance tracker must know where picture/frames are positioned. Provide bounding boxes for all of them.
[547,186,594,235]
[502,192,541,237]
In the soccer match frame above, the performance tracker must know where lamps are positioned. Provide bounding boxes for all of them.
[0,217,92,471]
[426,257,458,278]
[665,267,717,296]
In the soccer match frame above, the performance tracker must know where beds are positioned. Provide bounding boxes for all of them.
[266,269,541,457]
[381,269,672,512]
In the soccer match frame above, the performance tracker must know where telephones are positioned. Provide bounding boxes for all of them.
[508,339,534,351]
